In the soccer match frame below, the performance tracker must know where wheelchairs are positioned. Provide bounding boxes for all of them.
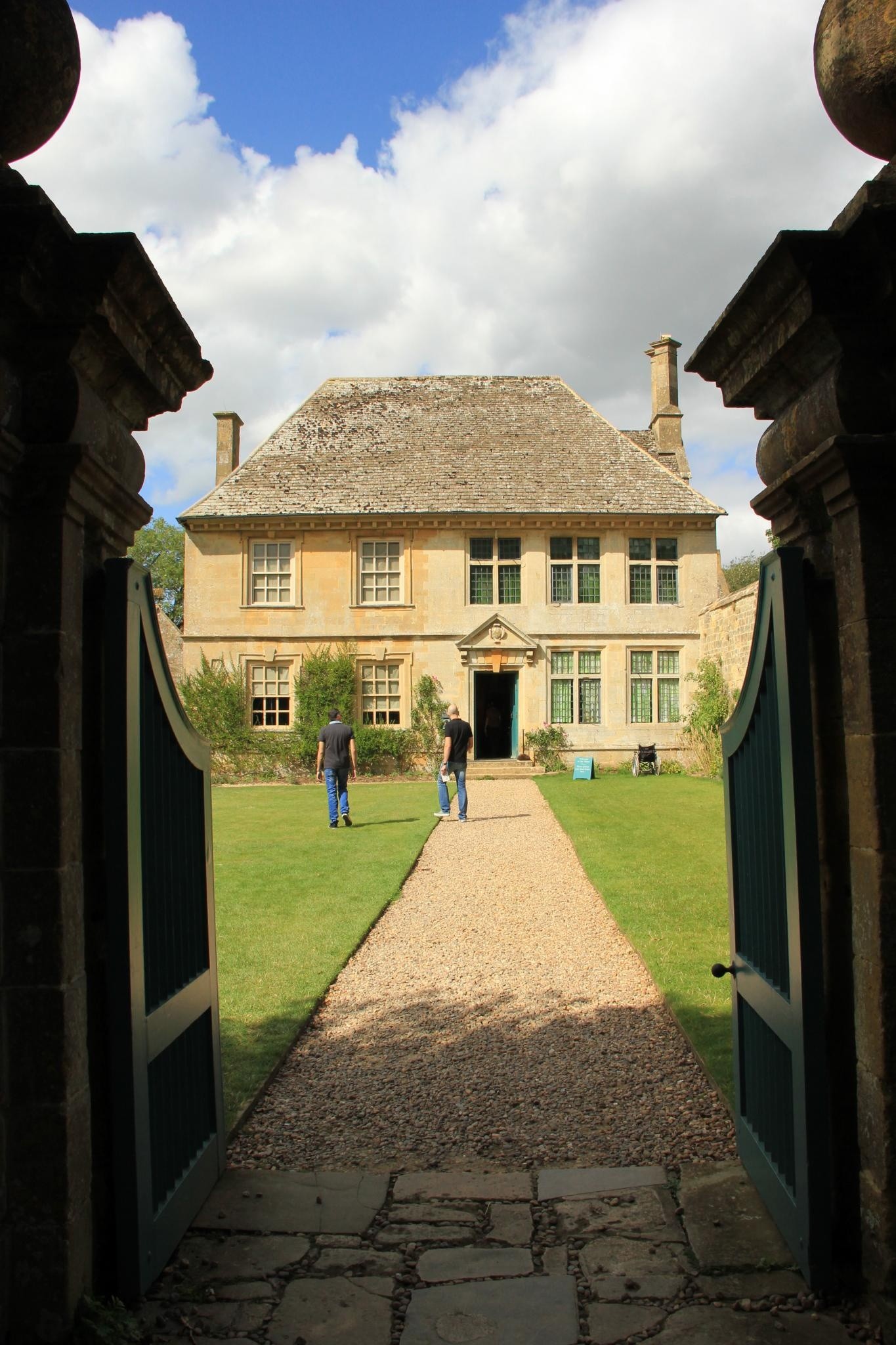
[632,743,661,777]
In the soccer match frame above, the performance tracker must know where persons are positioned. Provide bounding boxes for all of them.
[483,697,502,758]
[433,703,474,823]
[314,707,357,828]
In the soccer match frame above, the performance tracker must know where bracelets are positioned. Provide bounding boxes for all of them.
[316,770,321,772]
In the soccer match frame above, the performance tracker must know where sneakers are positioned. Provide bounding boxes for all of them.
[329,821,338,828]
[341,813,352,826]
[433,811,450,817]
[459,819,467,822]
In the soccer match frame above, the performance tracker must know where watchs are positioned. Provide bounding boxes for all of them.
[442,762,447,766]
